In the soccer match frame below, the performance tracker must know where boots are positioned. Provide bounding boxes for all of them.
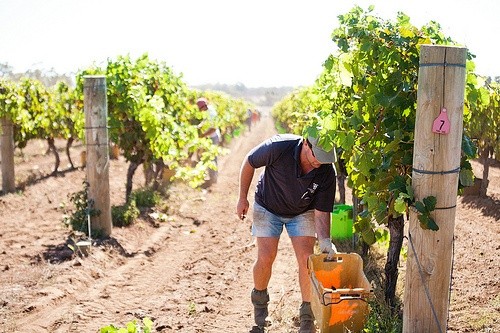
[250,288,273,326]
[298,301,317,333]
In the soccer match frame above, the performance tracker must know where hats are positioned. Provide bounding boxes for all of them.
[197,101,207,109]
[308,127,337,164]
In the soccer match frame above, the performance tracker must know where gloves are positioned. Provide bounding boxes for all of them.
[318,236,334,261]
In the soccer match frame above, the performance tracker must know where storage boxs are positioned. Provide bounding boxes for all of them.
[330,205,353,243]
[307,252,371,333]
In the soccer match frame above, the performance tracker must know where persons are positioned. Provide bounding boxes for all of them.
[246,108,260,132]
[196,101,221,189]
[237,135,336,333]
